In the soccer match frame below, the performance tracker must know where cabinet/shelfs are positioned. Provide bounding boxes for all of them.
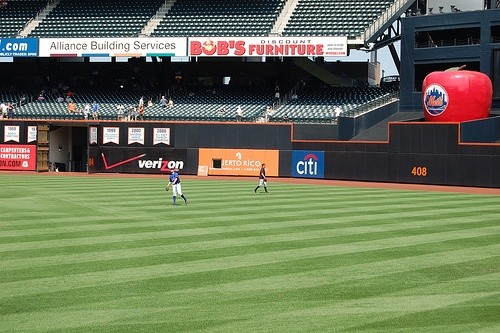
[37,122,50,172]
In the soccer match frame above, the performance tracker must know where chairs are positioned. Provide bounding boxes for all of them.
[0,84,400,124]
[0,0,397,40]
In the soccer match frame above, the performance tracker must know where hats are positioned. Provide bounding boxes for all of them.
[170,168,174,170]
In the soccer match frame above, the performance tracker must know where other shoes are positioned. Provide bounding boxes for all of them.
[253,189,256,194]
[265,192,270,194]
[185,198,188,202]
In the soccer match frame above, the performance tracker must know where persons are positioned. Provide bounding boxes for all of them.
[254,164,268,193]
[38,94,44,100]
[189,91,194,96]
[69,101,77,111]
[119,105,123,114]
[275,86,279,99]
[78,104,82,112]
[268,107,272,116]
[166,168,186,206]
[56,94,72,102]
[92,101,98,120]
[0,101,13,116]
[292,92,297,99]
[139,96,144,120]
[236,106,242,115]
[168,98,173,107]
[159,96,168,107]
[283,114,288,121]
[85,103,89,120]
[131,105,137,121]
[148,100,152,106]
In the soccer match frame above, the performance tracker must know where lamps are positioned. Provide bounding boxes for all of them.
[407,9,412,17]
[428,8,433,15]
[439,6,444,14]
[58,144,63,152]
[450,5,455,13]
[416,8,421,15]
